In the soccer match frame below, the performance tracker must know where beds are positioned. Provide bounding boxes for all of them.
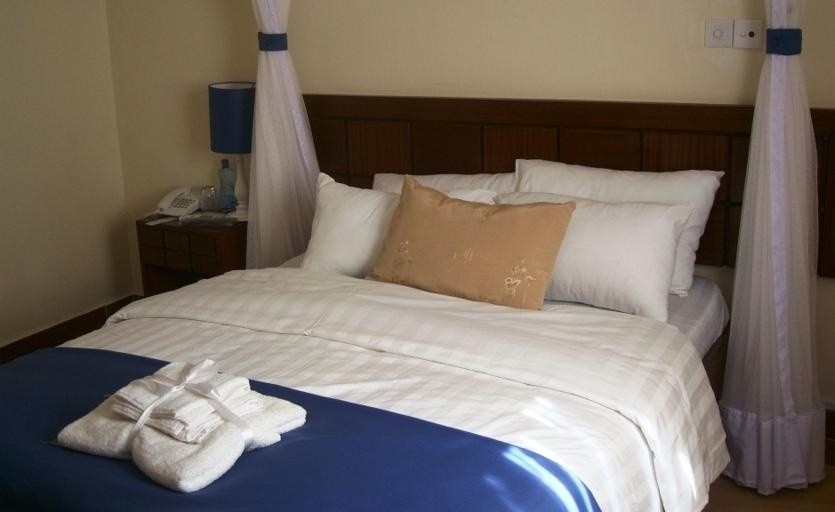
[0,243,731,512]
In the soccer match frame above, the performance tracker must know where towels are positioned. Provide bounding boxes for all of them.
[110,362,263,444]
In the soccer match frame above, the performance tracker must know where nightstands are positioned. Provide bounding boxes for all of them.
[135,211,248,297]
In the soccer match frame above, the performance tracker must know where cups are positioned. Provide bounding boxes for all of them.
[198,186,218,218]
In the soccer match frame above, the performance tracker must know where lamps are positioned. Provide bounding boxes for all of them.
[209,81,256,221]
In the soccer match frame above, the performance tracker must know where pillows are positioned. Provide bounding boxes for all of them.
[496,191,695,324]
[513,158,725,298]
[371,172,514,196]
[298,171,497,284]
[364,173,576,311]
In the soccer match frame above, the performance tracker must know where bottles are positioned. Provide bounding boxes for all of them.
[217,159,236,212]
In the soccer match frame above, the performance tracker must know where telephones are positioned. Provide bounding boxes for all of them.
[154,185,202,216]
